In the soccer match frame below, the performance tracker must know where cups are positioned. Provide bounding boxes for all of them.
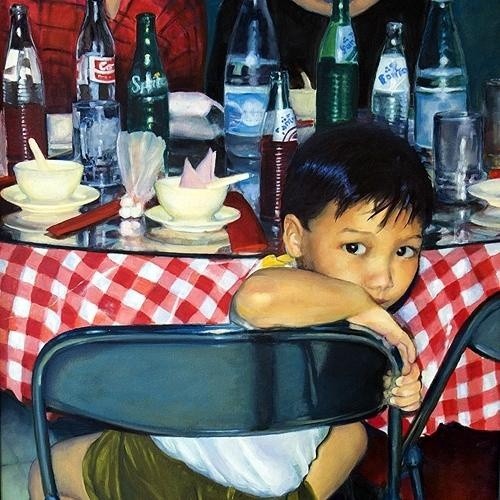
[436,109,484,202]
[19,232,78,246]
[483,78,500,171]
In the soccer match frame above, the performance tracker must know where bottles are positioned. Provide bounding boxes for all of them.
[0,1,468,228]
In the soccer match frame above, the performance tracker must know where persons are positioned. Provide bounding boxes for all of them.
[27,118,435,500]
[207,0,436,113]
[0,0,207,162]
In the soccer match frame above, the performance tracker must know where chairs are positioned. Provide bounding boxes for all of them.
[397,289,500,500]
[31,324,402,500]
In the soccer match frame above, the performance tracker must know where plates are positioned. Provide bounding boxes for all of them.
[467,177,499,209]
[470,207,499,229]
[148,227,231,249]
[0,212,85,232]
